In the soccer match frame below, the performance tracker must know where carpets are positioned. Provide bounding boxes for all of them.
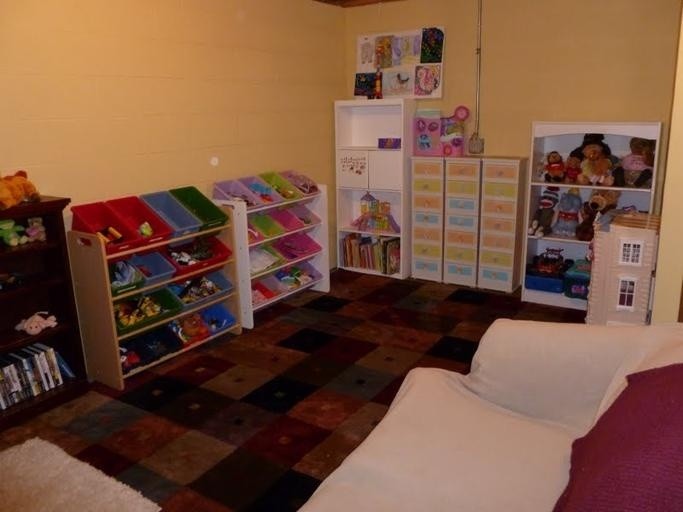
[1,435,163,512]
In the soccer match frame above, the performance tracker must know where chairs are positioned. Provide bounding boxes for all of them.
[297,318,683,512]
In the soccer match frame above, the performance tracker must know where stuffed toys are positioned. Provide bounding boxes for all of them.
[0,170,40,210]
[527,133,656,240]
[0,217,46,246]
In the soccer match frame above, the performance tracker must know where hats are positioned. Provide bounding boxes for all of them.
[542,185,559,200]
[580,134,610,153]
[571,147,584,161]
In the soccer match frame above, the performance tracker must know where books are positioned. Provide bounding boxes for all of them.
[339,233,400,275]
[0,343,75,410]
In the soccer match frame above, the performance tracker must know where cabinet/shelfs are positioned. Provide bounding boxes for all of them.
[520,121,662,309]
[66,206,241,389]
[410,154,524,293]
[333,100,414,279]
[210,185,327,334]
[1,192,87,430]
